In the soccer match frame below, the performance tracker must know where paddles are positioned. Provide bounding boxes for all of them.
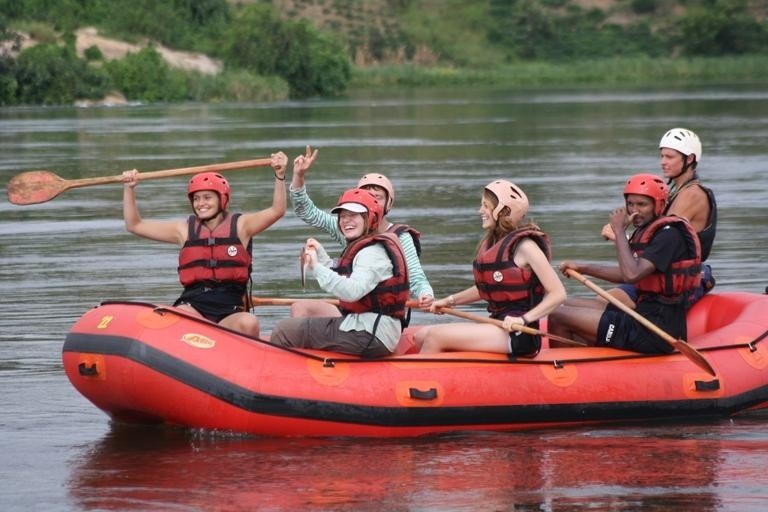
[8,156,280,205]
[242,295,427,308]
[556,262,717,377]
[430,304,587,346]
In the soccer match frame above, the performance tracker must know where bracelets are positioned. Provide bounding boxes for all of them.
[521,315,528,325]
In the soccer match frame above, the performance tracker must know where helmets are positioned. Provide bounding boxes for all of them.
[338,189,382,235]
[188,172,231,211]
[484,180,528,226]
[658,129,701,165]
[355,172,395,213]
[623,174,668,216]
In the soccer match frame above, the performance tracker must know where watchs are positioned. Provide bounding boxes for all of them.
[448,294,455,306]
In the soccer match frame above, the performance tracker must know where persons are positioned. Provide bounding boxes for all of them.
[271,188,409,359]
[547,174,701,353]
[413,179,567,358]
[601,127,717,310]
[289,146,435,328]
[122,152,288,338]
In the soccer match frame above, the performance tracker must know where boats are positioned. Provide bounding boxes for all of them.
[62,292,768,439]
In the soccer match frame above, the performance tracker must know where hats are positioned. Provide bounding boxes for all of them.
[332,203,368,215]
